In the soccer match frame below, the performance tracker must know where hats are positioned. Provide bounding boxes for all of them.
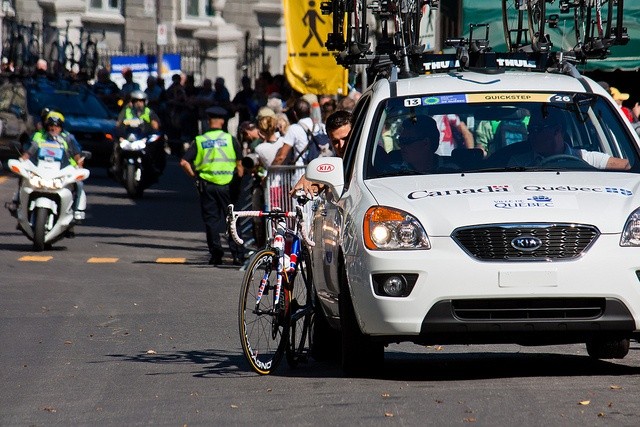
[206,106,228,120]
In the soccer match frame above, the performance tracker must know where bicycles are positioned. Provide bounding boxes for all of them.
[2,16,105,78]
[226,204,312,375]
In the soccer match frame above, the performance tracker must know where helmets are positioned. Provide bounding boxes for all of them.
[45,112,65,124]
[130,91,149,107]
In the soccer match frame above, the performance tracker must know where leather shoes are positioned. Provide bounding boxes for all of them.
[232,258,244,265]
[209,258,223,264]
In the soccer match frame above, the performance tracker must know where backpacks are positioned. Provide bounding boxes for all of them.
[294,122,335,166]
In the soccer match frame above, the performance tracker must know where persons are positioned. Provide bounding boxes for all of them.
[108,90,172,175]
[4,105,87,225]
[325,112,394,177]
[434,110,531,156]
[504,105,631,175]
[374,114,460,179]
[179,106,247,267]
[1,54,201,126]
[237,97,329,160]
[202,69,363,138]
[595,80,639,140]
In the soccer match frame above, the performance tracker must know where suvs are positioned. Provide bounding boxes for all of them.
[1,80,117,166]
[305,62,640,370]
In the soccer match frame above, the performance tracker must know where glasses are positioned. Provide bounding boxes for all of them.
[396,138,426,145]
[47,116,60,125]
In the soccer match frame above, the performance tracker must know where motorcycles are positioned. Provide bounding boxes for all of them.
[106,120,162,199]
[5,141,92,251]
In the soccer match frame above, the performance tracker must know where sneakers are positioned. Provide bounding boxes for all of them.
[6,202,16,210]
[74,211,84,220]
